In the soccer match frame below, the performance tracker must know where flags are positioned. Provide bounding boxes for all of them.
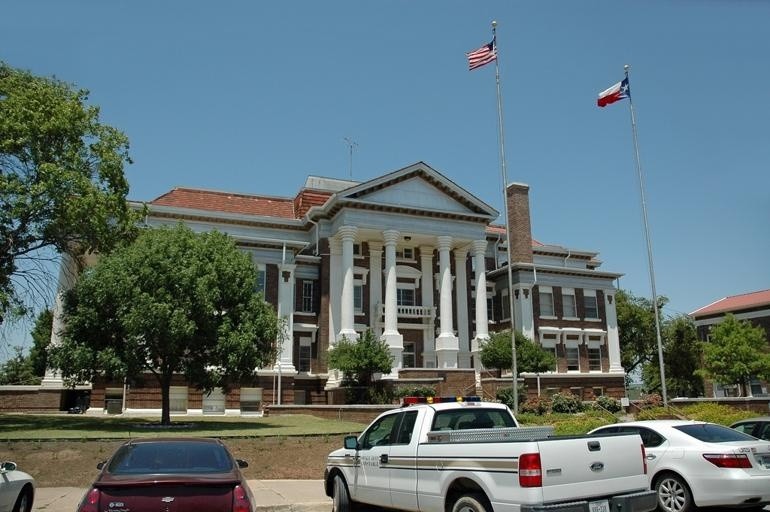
[466,38,495,71]
[596,79,630,108]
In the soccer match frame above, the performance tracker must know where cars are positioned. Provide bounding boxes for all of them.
[0,462,36,512]
[583,419,770,511]
[76,438,256,512]
[730,418,770,439]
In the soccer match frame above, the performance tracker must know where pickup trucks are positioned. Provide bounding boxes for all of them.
[322,397,661,511]
